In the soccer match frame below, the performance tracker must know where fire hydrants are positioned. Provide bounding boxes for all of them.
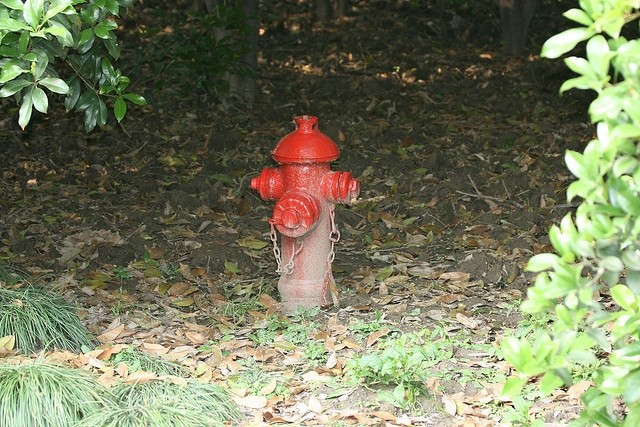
[250,114,361,313]
